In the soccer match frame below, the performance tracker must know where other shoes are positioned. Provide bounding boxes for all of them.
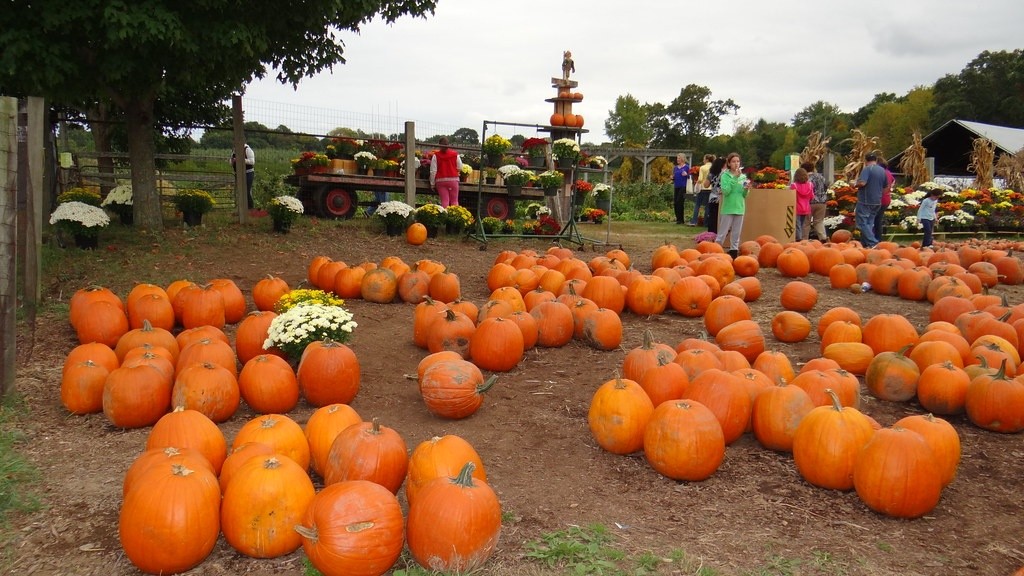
[674,221,684,224]
[687,223,696,226]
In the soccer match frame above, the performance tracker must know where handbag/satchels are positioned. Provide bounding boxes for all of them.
[685,175,694,194]
[695,181,701,193]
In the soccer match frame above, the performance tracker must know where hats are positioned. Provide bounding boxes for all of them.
[865,152,874,158]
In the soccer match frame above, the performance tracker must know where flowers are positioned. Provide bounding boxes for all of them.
[49,184,133,236]
[267,136,614,236]
[695,231,717,243]
[172,189,216,213]
[741,167,1024,244]
[262,288,359,363]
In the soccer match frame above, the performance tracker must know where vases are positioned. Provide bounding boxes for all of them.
[74,234,98,250]
[274,218,290,233]
[485,230,493,234]
[465,228,476,234]
[571,195,585,205]
[597,199,609,209]
[574,216,579,221]
[387,225,401,236]
[360,167,368,175]
[120,212,133,225]
[508,185,522,196]
[460,176,467,182]
[373,169,385,176]
[581,217,587,222]
[446,223,459,235]
[486,177,496,184]
[595,220,602,223]
[558,156,574,168]
[427,227,438,237]
[183,211,202,227]
[488,153,503,166]
[543,185,558,196]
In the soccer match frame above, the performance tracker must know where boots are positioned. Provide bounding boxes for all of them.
[729,250,737,259]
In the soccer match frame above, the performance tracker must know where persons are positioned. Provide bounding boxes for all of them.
[715,152,750,259]
[704,157,727,234]
[801,162,829,243]
[855,152,888,248]
[686,154,716,227]
[562,50,575,78]
[429,138,463,209]
[917,188,943,247]
[790,168,815,241]
[670,153,690,224]
[873,149,895,241]
[231,135,255,209]
[363,191,387,217]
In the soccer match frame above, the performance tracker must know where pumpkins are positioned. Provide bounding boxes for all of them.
[308,230,1024,519]
[407,222,427,245]
[62,273,499,575]
[550,113,584,127]
[559,92,583,99]
